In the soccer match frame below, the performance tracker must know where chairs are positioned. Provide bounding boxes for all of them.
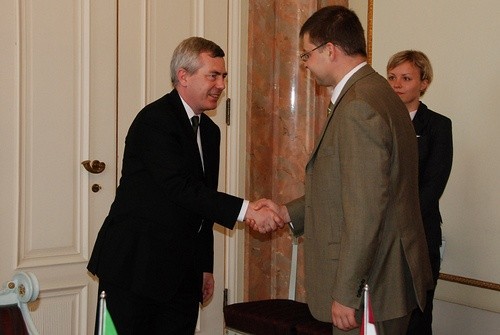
[223,235,333,335]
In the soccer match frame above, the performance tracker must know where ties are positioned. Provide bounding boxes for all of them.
[191,116,199,142]
[327,100,334,119]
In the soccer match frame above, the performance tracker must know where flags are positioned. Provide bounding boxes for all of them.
[98,298,118,335]
[359,290,377,335]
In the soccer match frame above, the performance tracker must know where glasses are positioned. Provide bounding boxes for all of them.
[299,42,338,62]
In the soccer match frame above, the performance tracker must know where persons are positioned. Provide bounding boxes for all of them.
[386,50,454,335]
[245,4,435,335]
[86,37,285,335]
